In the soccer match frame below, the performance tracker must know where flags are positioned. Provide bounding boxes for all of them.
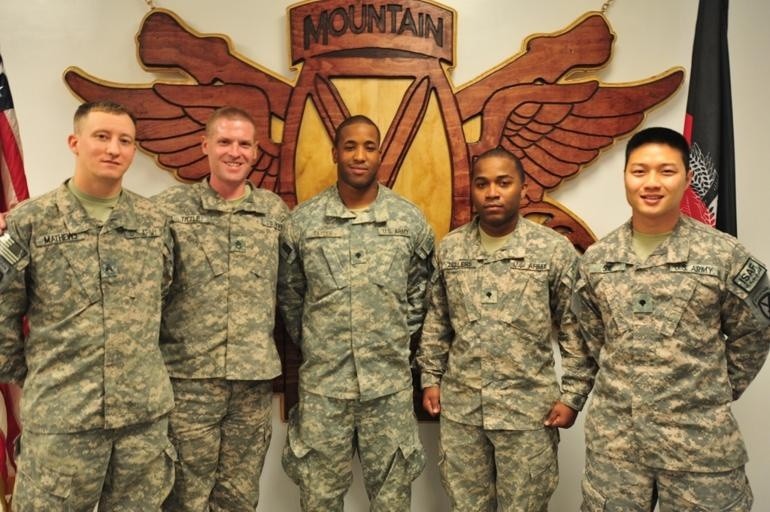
[681,0,740,244]
[0,55,30,511]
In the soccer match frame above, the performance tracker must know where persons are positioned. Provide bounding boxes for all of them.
[0,105,293,511]
[278,113,439,512]
[414,147,597,511]
[0,100,179,511]
[569,128,770,511]
